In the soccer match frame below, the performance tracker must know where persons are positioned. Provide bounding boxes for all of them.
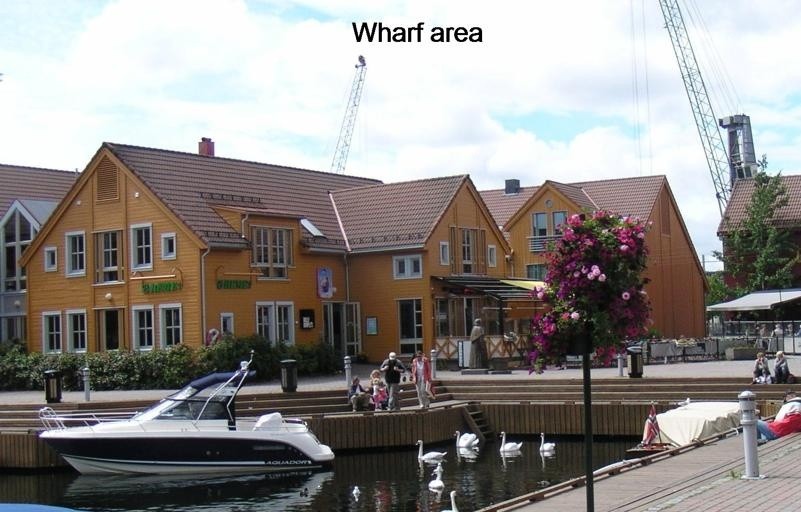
[773,351,789,383]
[365,370,389,410]
[759,324,801,337]
[410,350,432,409]
[379,352,405,412]
[372,378,382,410]
[751,352,771,384]
[471,318,489,368]
[757,392,801,440]
[348,376,371,412]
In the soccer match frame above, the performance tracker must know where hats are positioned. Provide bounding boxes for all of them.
[417,350,423,357]
[389,351,397,361]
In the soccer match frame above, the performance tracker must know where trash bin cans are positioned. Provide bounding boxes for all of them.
[44,370,62,403]
[625,346,644,378]
[280,359,298,392]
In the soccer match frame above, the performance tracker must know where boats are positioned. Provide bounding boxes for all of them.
[38,365,337,476]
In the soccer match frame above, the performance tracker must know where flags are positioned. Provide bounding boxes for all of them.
[641,404,661,449]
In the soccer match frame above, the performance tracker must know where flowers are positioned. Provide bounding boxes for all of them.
[528,208,653,373]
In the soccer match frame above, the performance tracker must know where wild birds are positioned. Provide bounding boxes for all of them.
[436,463,442,468]
[299,488,309,495]
[352,484,361,499]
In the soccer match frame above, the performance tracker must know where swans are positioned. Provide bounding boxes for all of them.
[440,491,459,512]
[428,468,446,490]
[499,431,523,453]
[452,428,478,448]
[414,438,447,460]
[540,430,556,453]
